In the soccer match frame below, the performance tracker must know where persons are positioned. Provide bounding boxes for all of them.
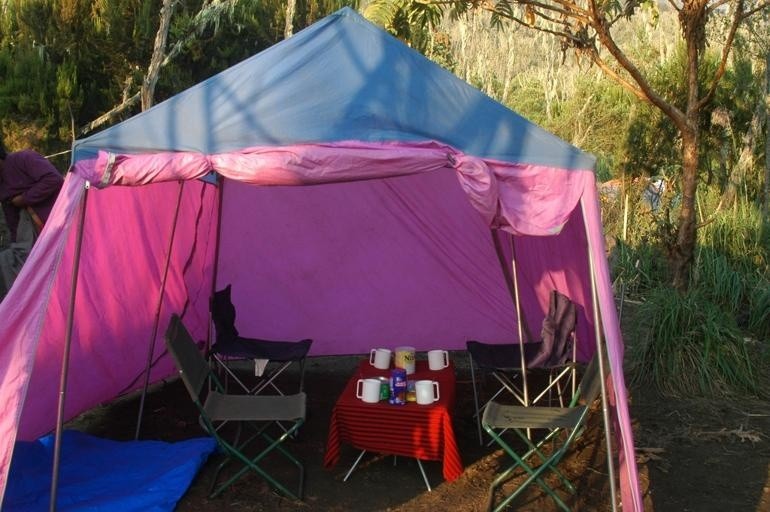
[0,138,65,267]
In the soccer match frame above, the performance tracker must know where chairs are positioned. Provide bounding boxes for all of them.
[163,313,314,502]
[198,284,312,441]
[467,288,584,442]
[480,339,615,512]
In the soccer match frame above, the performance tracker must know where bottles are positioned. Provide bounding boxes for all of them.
[374,368,406,406]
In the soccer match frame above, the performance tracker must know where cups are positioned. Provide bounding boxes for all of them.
[368,347,392,370]
[427,349,450,371]
[415,380,442,405]
[355,378,380,403]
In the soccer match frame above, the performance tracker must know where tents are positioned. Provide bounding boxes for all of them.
[2,4,644,511]
